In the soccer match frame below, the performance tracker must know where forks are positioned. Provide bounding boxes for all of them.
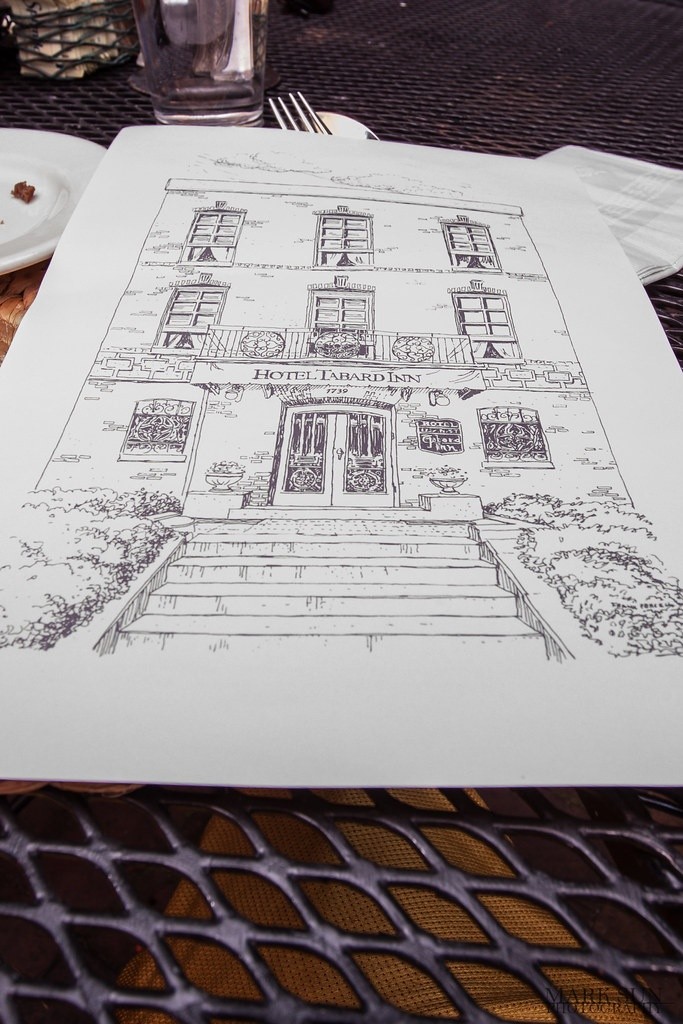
[268,91,333,136]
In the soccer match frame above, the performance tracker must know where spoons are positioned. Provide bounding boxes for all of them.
[293,110,380,141]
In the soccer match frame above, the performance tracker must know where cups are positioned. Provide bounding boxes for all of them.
[131,0,269,129]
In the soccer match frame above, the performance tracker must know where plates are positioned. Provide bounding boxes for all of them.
[0,126,108,276]
